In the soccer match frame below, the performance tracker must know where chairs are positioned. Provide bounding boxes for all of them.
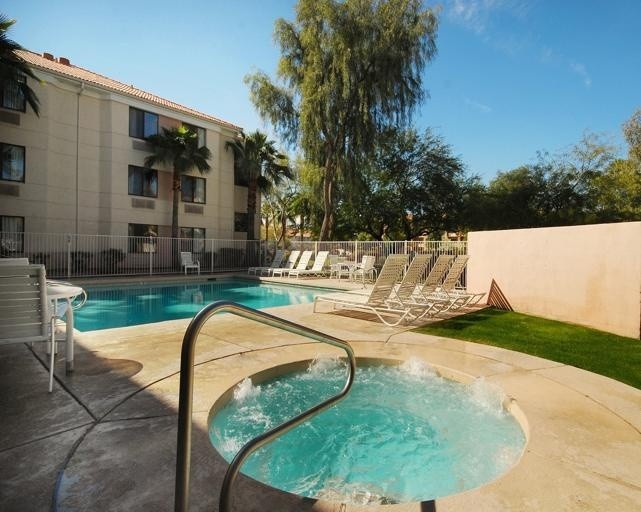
[180,252,201,276]
[247,249,487,327]
[0,258,87,393]
